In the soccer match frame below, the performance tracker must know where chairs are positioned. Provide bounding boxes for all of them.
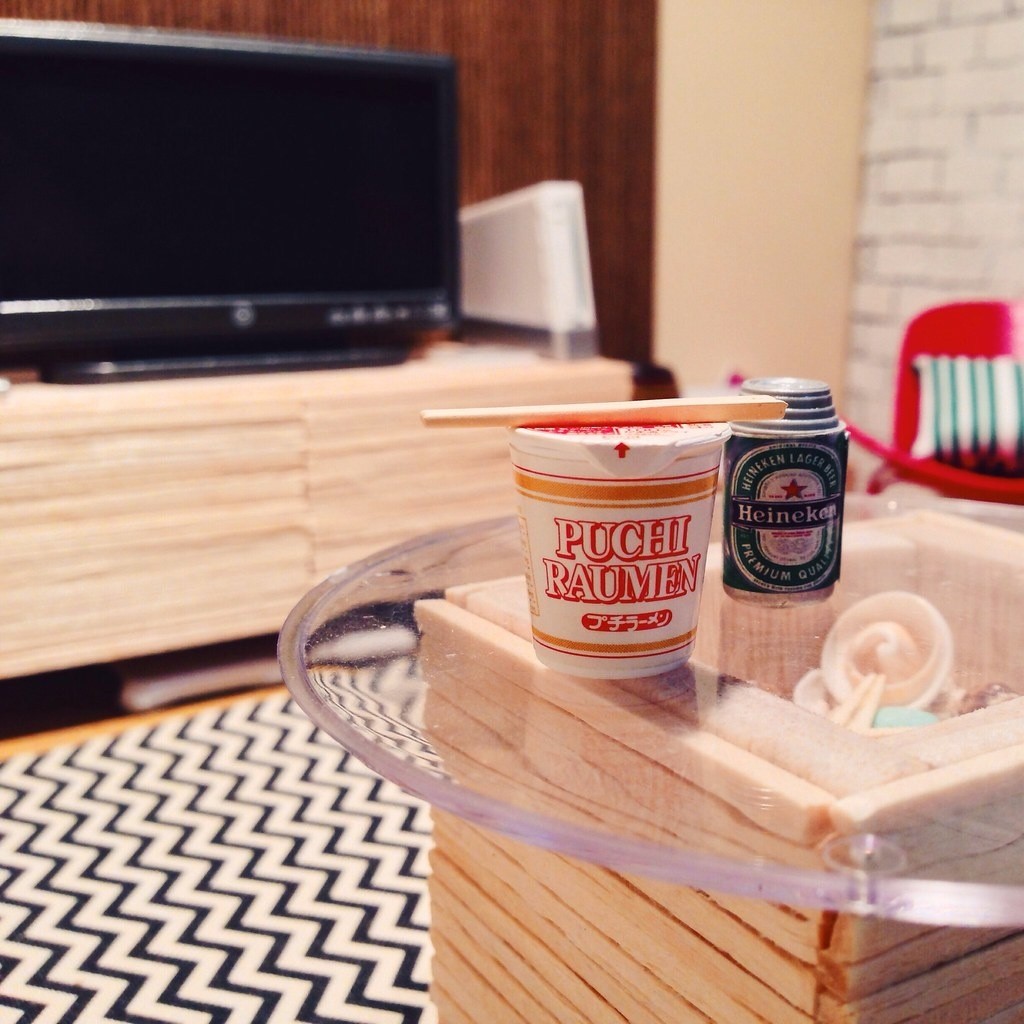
[859,299,1024,509]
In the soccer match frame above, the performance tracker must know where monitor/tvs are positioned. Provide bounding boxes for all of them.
[0,18,461,385]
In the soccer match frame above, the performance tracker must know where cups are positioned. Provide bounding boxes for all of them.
[506,423,734,681]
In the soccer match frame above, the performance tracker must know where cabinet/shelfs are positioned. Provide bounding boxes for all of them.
[1,328,636,688]
